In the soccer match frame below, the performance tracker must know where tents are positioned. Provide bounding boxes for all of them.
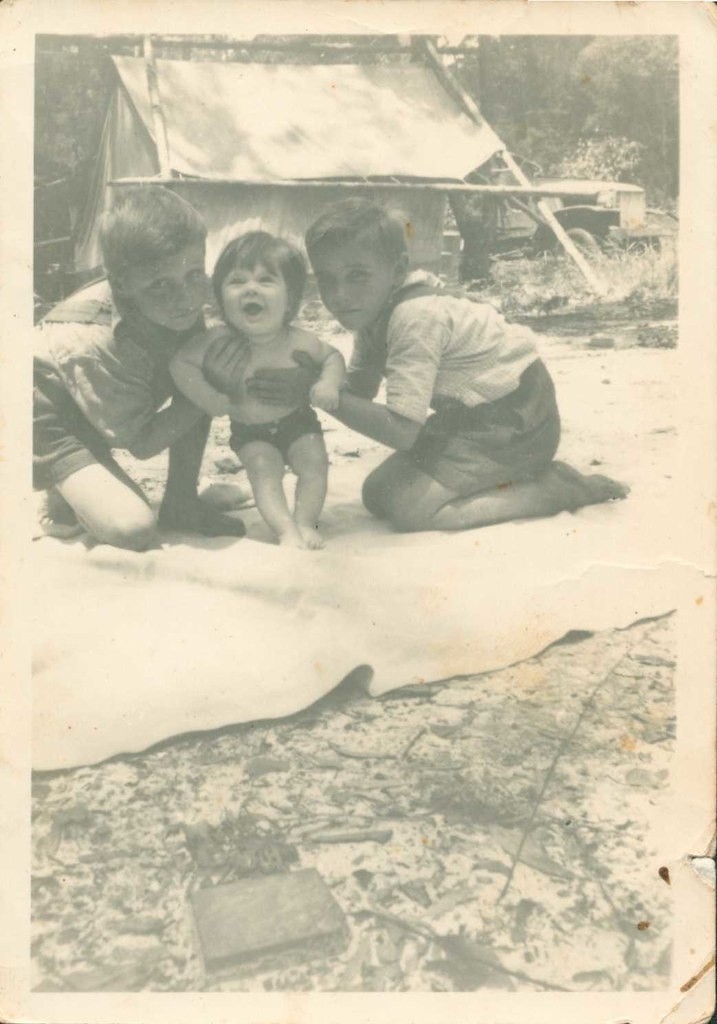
[63,53,600,283]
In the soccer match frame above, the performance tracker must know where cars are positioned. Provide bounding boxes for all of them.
[497,153,646,257]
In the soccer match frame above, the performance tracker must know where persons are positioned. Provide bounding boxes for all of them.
[168,232,346,549]
[33,184,253,548]
[244,198,631,533]
